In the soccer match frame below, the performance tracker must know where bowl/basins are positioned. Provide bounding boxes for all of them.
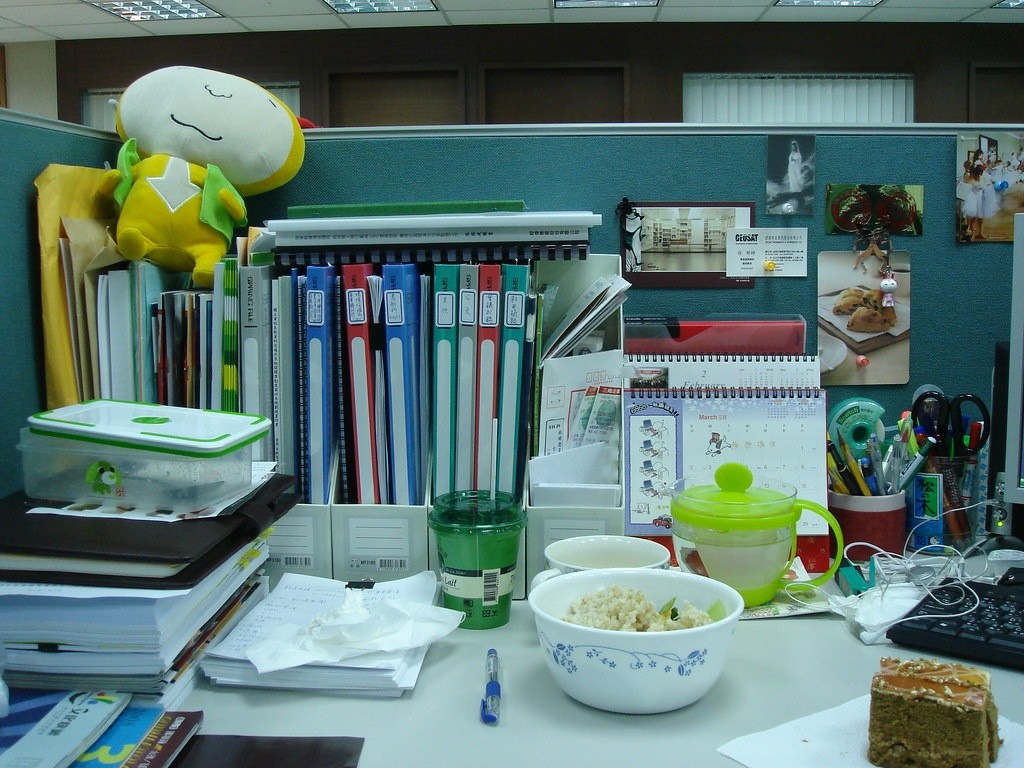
[528,566,745,714]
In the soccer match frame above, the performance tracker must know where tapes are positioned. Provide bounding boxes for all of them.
[828,397,884,459]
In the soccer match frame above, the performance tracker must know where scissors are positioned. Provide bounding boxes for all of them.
[913,390,993,480]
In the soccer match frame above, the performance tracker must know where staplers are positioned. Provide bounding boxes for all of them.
[834,556,878,598]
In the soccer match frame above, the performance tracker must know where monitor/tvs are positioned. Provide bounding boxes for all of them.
[1003,212,1024,505]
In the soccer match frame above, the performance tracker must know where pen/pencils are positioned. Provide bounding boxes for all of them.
[827,411,991,555]
[482,648,501,723]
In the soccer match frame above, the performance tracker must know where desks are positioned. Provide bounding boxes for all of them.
[175,574,1024,768]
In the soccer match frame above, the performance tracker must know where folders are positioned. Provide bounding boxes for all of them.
[306,263,532,506]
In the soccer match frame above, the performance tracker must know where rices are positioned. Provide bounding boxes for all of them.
[558,584,715,633]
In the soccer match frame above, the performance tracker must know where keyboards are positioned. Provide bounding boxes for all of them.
[887,577,1024,669]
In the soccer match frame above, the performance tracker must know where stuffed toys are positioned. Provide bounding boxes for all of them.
[109,65,316,289]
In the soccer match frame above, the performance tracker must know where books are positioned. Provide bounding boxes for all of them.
[0,202,298,768]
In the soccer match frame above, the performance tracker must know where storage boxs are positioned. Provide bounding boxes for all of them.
[14,397,276,510]
[262,441,338,591]
[330,424,432,583]
[521,253,629,600]
[428,434,527,601]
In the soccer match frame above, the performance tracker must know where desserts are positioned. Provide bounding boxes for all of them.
[866,655,1004,768]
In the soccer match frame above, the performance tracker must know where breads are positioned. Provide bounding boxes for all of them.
[832,288,897,333]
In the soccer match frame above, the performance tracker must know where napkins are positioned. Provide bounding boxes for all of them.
[713,692,1024,768]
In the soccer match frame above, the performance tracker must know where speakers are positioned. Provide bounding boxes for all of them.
[985,342,1021,536]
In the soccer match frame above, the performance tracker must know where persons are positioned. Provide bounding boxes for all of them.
[789,143,804,192]
[959,145,1024,239]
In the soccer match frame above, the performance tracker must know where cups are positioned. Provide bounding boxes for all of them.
[906,455,979,555]
[531,535,672,590]
[427,489,529,630]
[826,483,907,562]
[669,462,844,606]
